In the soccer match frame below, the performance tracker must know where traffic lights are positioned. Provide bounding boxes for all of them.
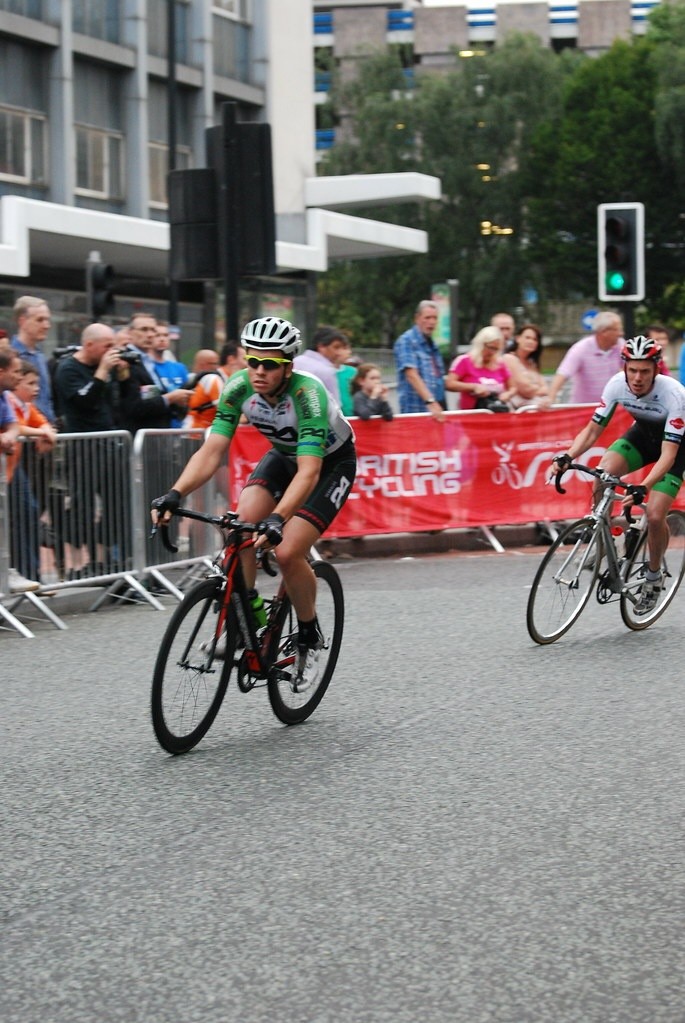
[596,203,645,302]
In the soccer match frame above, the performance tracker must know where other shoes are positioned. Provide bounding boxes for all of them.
[7,567,40,593]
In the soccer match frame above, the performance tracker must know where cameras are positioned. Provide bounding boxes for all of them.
[118,349,142,365]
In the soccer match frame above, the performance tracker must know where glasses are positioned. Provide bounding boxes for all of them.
[133,327,154,331]
[484,344,499,351]
[245,353,292,370]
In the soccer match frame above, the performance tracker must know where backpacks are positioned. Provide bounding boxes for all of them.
[169,369,227,412]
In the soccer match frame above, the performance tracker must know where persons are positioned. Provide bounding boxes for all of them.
[0,294,685,605]
[550,336,685,615]
[150,317,357,692]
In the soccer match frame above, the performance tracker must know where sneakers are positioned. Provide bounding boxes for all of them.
[290,624,325,693]
[204,628,244,656]
[633,569,664,615]
[573,540,608,568]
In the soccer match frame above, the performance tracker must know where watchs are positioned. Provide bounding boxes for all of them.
[424,397,436,404]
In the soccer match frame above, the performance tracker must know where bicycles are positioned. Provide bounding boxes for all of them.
[526,453,685,645]
[149,505,345,757]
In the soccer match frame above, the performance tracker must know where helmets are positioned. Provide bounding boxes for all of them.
[240,317,302,359]
[620,334,662,362]
